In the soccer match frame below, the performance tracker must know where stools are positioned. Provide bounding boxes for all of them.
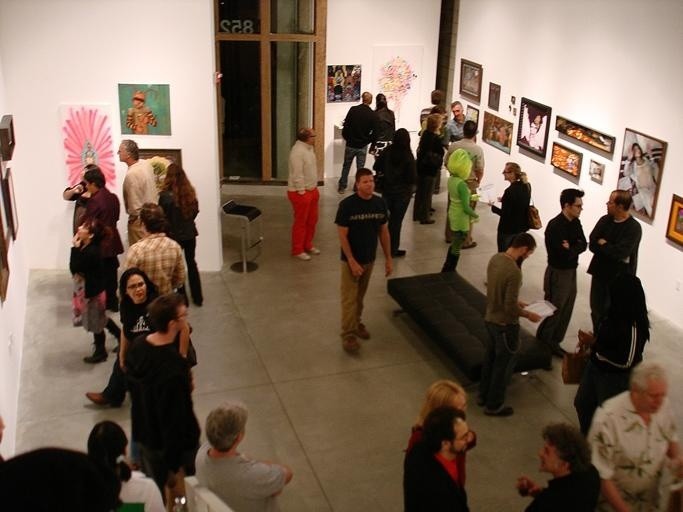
[221,197,269,274]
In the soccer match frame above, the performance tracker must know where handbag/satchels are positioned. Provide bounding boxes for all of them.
[560,352,593,386]
[528,204,543,231]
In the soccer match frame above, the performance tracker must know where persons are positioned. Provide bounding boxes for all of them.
[125,293,200,511]
[86,419,165,511]
[85,201,187,407]
[192,400,292,511]
[61,139,204,364]
[126,92,156,135]
[116,268,192,373]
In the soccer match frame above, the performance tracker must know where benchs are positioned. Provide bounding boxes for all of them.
[387,272,554,391]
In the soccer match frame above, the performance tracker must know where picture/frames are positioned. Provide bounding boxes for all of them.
[665,195,683,247]
[138,148,183,168]
[449,54,667,227]
[0,112,22,305]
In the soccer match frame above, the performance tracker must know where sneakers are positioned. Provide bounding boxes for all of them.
[303,247,320,255]
[355,323,373,342]
[341,333,364,354]
[337,186,479,250]
[290,250,312,262]
[551,345,570,359]
[477,391,515,419]
[182,297,204,309]
[387,248,407,259]
[81,349,108,366]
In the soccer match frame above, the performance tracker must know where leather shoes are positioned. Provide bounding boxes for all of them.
[84,389,126,410]
